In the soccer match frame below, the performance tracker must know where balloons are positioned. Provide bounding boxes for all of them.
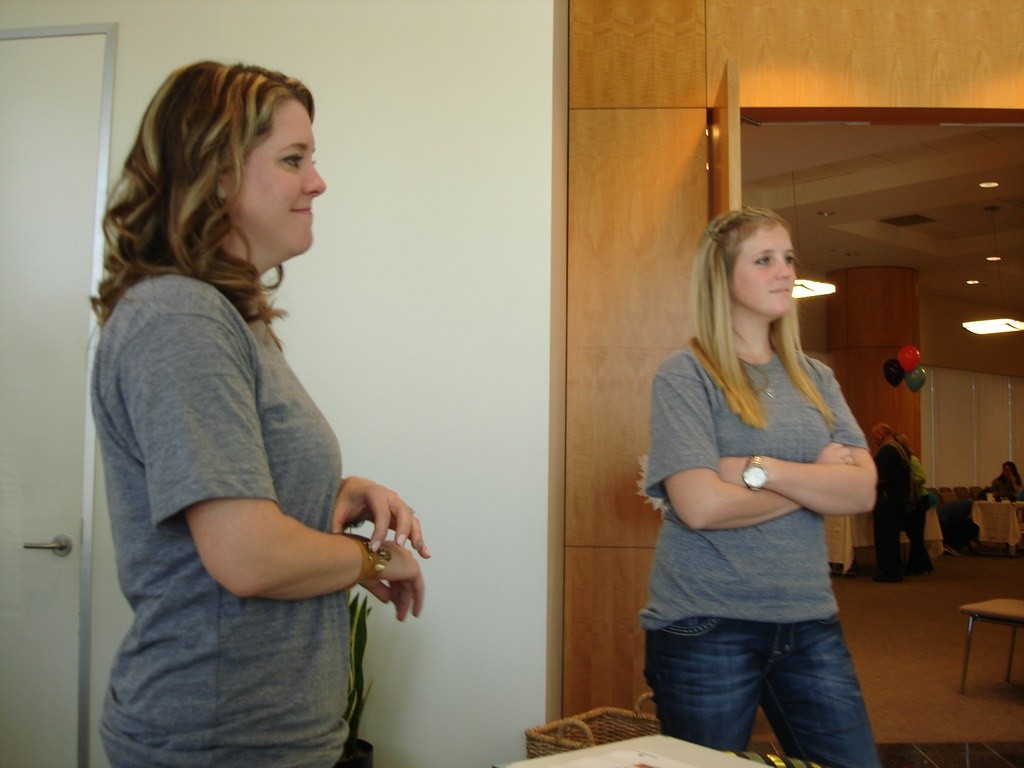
[882,345,926,395]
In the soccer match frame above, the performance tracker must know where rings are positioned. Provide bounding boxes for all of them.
[406,507,418,518]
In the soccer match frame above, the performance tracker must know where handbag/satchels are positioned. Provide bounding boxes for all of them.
[956,496,973,511]
[917,487,945,508]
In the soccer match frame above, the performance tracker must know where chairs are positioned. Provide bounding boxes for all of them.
[923,486,994,506]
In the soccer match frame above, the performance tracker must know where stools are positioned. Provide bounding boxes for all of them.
[958,597,1024,695]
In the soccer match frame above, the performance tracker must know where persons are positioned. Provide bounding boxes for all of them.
[88,58,430,766]
[990,459,1023,505]
[639,203,882,766]
[871,422,912,584]
[894,430,937,579]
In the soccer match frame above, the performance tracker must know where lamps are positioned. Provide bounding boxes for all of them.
[792,172,836,299]
[962,205,1024,334]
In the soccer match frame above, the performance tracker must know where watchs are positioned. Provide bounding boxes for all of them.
[742,455,771,492]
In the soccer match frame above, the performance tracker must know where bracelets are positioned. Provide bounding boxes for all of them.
[336,525,393,587]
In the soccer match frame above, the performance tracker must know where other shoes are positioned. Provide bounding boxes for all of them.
[917,563,933,571]
[872,570,903,583]
[902,567,918,576]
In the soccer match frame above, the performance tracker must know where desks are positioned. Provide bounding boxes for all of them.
[824,508,945,578]
[492,734,773,768]
[971,501,1024,557]
